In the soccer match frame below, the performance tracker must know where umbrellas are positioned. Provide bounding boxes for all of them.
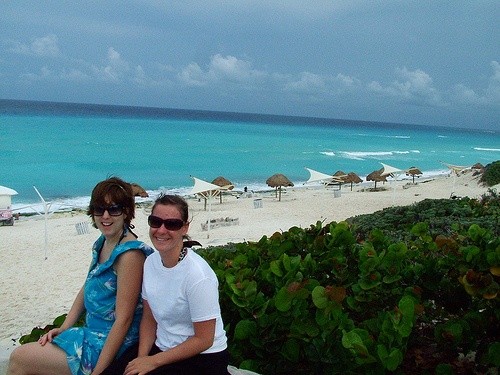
[187,161,490,239]
[125,182,149,218]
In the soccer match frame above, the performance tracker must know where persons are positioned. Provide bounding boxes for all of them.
[112,194,229,375]
[6,176,154,375]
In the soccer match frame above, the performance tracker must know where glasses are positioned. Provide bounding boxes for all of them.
[147,215,186,231]
[91,204,123,216]
